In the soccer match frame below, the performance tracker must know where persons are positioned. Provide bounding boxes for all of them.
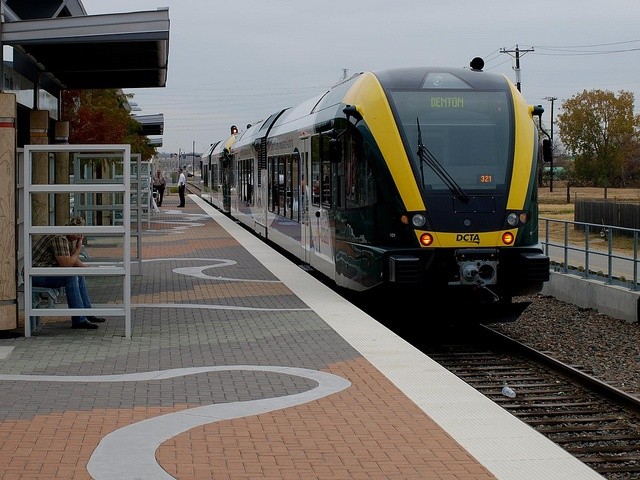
[20,216,106,330]
[147,170,166,206]
[176,168,186,207]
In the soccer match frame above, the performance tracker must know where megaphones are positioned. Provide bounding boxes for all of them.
[470,57,484,70]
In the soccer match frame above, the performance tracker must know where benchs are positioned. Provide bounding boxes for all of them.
[17,283,65,331]
[110,209,123,222]
[77,245,88,261]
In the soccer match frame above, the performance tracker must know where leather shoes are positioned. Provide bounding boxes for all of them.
[88,316,107,323]
[177,205,185,207]
[72,321,101,329]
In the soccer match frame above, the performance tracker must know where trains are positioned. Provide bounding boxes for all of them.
[200,55,553,323]
